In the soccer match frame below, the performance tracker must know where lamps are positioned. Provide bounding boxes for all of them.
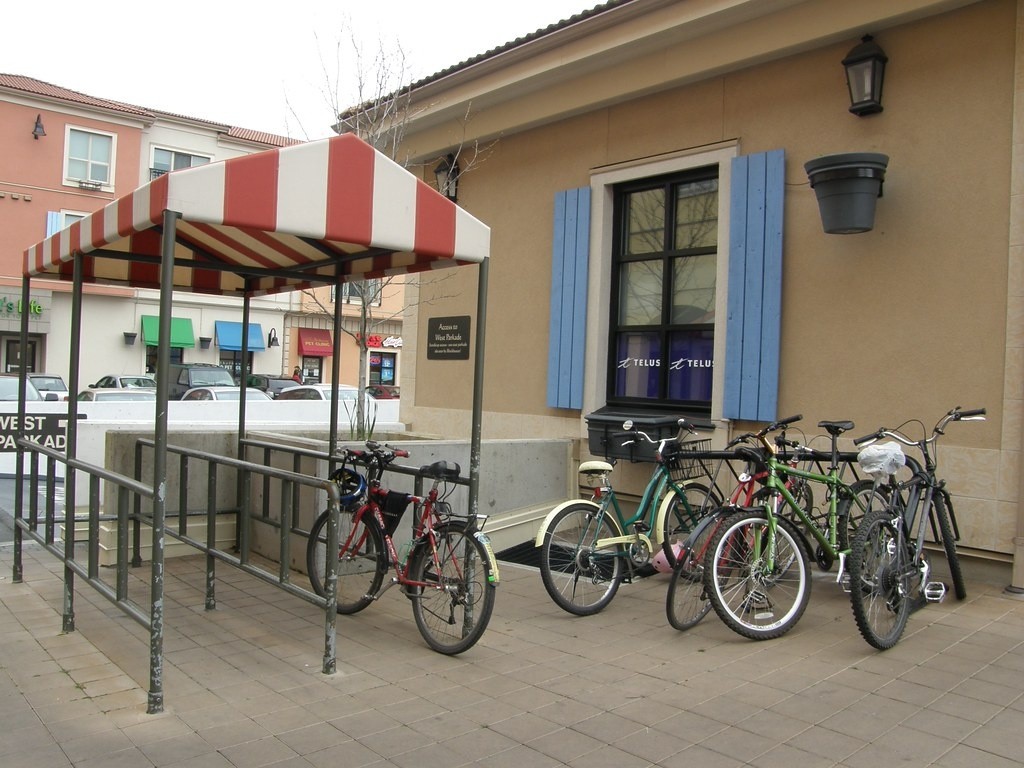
[267,328,279,348]
[839,32,889,118]
[433,151,461,205]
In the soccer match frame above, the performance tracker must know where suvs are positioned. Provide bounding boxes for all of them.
[278,382,376,400]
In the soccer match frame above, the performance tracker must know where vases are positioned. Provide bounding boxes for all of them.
[801,152,890,234]
[585,411,680,466]
[124,332,137,345]
[200,336,212,350]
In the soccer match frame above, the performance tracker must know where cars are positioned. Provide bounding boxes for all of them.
[248,373,305,399]
[365,385,401,399]
[0,371,44,402]
[88,375,157,389]
[77,387,157,402]
[180,387,274,402]
[26,373,71,402]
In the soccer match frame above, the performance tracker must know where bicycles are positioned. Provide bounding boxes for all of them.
[663,430,833,634]
[702,413,906,640]
[304,440,501,657]
[534,417,733,616]
[841,406,991,653]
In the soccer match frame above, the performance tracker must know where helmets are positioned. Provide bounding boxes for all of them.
[650,539,692,573]
[327,450,367,506]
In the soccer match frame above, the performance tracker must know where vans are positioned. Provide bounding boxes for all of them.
[167,362,237,400]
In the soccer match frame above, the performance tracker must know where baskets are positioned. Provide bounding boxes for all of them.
[654,437,715,484]
